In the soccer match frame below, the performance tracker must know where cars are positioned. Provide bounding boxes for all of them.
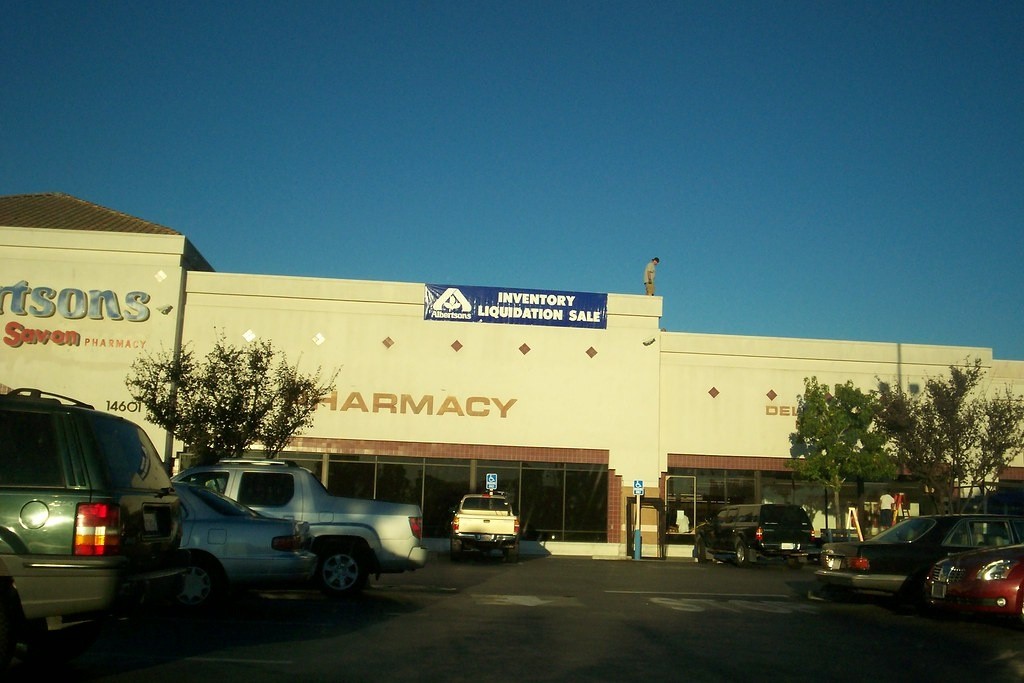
[170,480,318,620]
[813,515,1024,615]
[923,544,1024,629]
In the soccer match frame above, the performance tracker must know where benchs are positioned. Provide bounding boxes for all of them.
[820,529,859,543]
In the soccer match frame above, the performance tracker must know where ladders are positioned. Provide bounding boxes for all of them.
[886,493,910,528]
[846,507,863,542]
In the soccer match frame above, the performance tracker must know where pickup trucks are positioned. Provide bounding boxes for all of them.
[449,493,521,564]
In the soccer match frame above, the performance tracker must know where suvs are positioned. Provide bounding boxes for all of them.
[695,503,815,570]
[170,458,429,602]
[1,389,187,683]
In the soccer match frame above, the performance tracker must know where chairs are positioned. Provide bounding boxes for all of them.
[956,532,1004,546]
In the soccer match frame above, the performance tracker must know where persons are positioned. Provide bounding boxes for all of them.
[643,258,659,296]
[878,490,896,531]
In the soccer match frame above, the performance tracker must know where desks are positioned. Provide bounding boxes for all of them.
[535,528,607,542]
